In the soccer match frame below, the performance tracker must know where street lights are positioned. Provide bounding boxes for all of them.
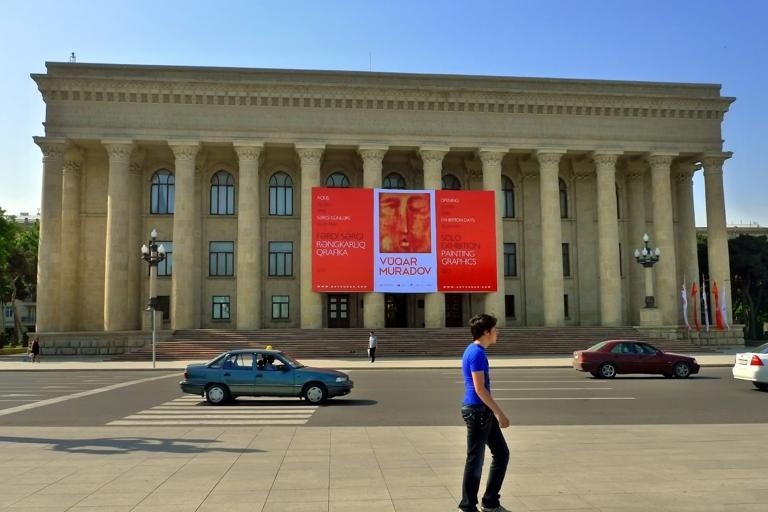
[140,228,165,311]
[633,231,662,309]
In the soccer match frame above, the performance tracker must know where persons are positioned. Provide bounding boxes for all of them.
[31,335,41,363]
[368,331,377,363]
[264,355,278,371]
[380,194,431,253]
[456,314,514,512]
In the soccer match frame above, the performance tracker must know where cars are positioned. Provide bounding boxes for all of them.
[179,345,355,406]
[572,339,701,379]
[730,339,768,390]
